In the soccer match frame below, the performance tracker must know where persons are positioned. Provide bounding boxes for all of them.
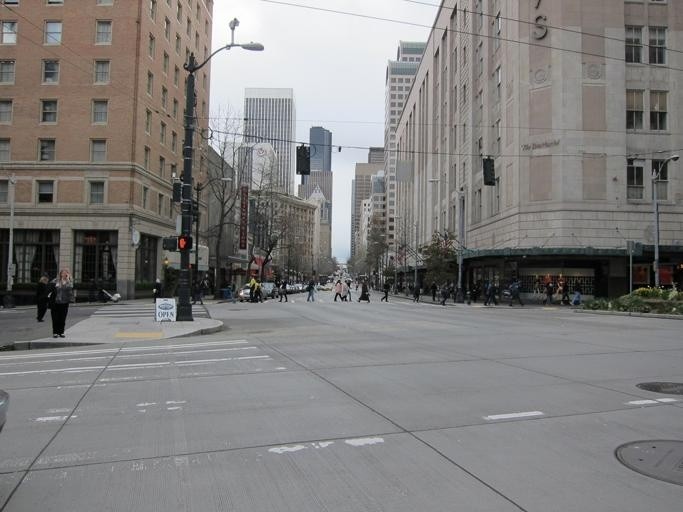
[380,280,391,302]
[555,272,565,294]
[542,286,548,307]
[532,274,540,295]
[204,273,210,285]
[192,282,204,305]
[356,281,371,303]
[347,280,354,302]
[307,280,316,303]
[152,277,162,304]
[247,275,258,303]
[542,272,552,293]
[546,282,556,304]
[278,280,289,303]
[35,276,51,321]
[341,280,349,302]
[570,280,585,307]
[408,278,526,307]
[49,268,77,338]
[561,282,573,306]
[254,285,266,304]
[334,279,344,302]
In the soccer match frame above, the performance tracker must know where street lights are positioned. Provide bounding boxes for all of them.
[175,40,264,323]
[374,216,418,297]
[650,154,680,287]
[428,179,464,303]
[192,175,233,301]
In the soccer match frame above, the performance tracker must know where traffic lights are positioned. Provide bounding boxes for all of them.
[177,236,192,250]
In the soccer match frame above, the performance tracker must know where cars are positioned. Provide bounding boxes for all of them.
[236,269,351,303]
